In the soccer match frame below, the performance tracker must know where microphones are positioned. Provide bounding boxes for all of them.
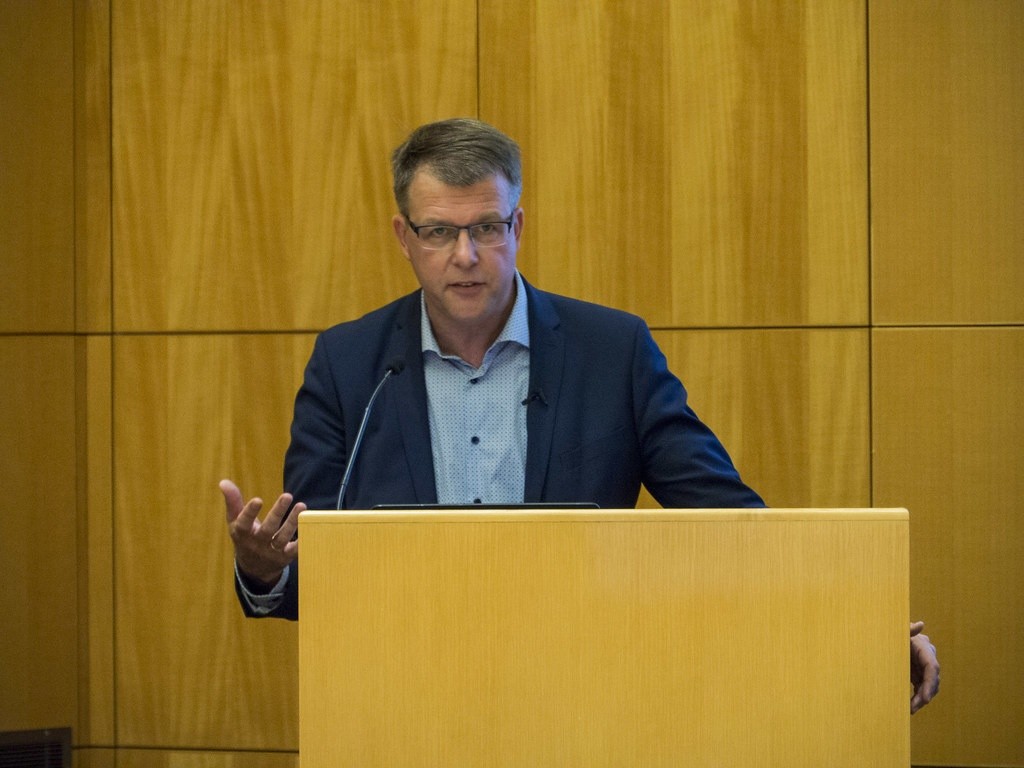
[521,392,538,405]
[337,354,408,510]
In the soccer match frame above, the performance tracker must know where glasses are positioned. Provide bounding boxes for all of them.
[406,210,514,250]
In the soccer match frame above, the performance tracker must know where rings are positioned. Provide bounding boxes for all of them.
[270,542,285,553]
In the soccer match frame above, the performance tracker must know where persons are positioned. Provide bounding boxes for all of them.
[218,118,939,717]
